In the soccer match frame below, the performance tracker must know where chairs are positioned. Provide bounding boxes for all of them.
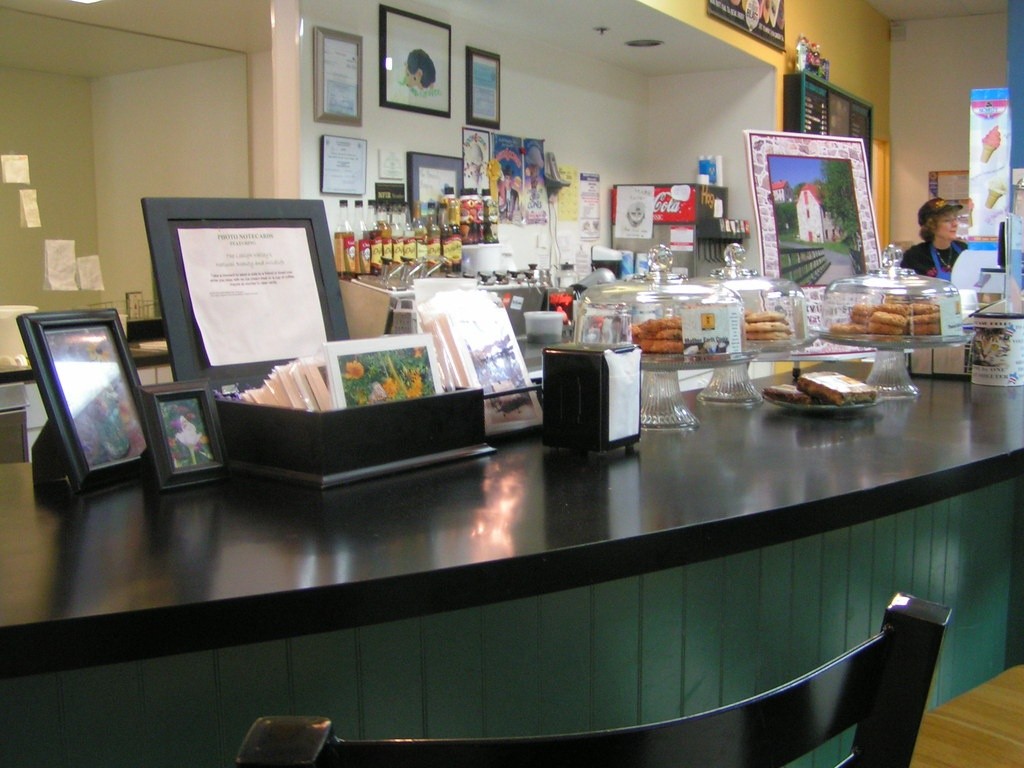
[235,590,954,768]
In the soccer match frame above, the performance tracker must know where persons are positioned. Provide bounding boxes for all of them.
[498,165,520,221]
[900,197,968,282]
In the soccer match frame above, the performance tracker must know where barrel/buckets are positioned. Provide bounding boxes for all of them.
[460,244,501,272]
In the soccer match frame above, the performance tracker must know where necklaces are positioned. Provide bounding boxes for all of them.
[936,245,952,270]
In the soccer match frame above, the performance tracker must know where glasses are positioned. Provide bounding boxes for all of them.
[935,217,958,224]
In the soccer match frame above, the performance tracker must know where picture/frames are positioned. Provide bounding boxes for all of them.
[323,334,443,411]
[16,309,148,498]
[134,376,231,493]
[465,45,501,130]
[312,24,363,126]
[378,3,452,118]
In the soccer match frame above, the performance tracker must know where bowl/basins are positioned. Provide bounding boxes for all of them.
[523,310,564,345]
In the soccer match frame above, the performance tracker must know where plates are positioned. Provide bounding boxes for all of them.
[763,390,884,415]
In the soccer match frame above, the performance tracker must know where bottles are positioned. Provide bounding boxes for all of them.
[562,262,576,287]
[796,36,827,78]
[335,186,501,273]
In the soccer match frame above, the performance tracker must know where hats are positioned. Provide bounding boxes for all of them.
[918,199,963,227]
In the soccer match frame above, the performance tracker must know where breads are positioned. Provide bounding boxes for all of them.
[743,310,794,340]
[829,302,940,336]
[763,371,877,406]
[632,315,684,354]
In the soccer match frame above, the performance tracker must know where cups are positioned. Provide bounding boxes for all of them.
[619,251,649,277]
[971,313,1024,386]
[576,301,633,346]
